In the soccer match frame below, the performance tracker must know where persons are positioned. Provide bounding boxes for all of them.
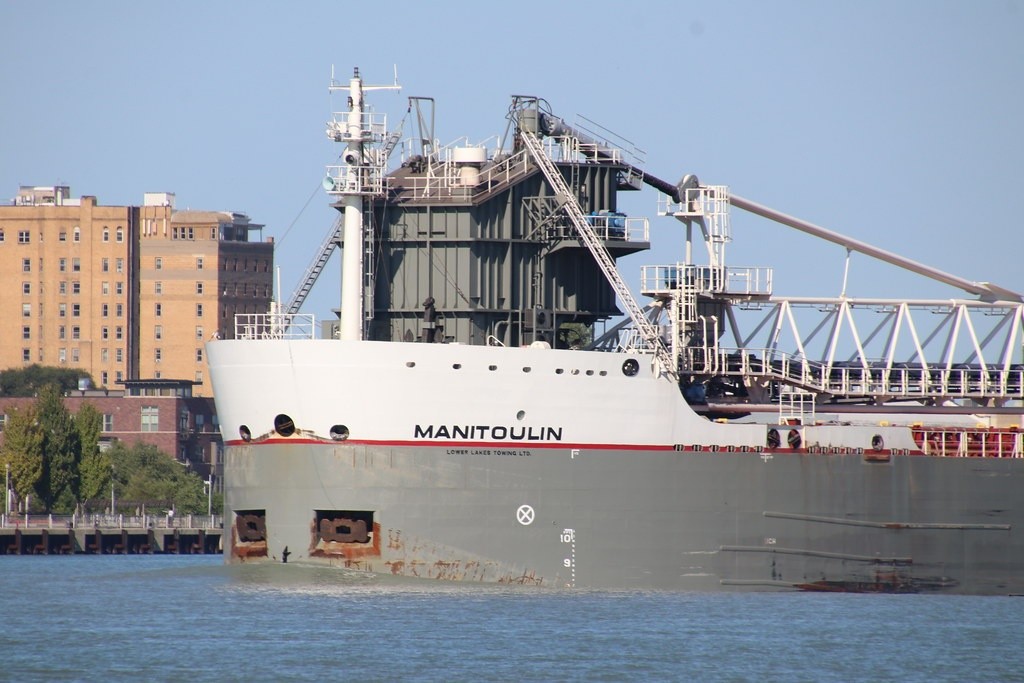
[678,373,728,406]
[163,507,175,526]
[530,335,550,350]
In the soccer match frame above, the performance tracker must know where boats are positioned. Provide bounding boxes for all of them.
[201,58,1024,595]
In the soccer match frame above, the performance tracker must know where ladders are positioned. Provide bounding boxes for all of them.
[270,132,402,338]
[521,129,672,369]
